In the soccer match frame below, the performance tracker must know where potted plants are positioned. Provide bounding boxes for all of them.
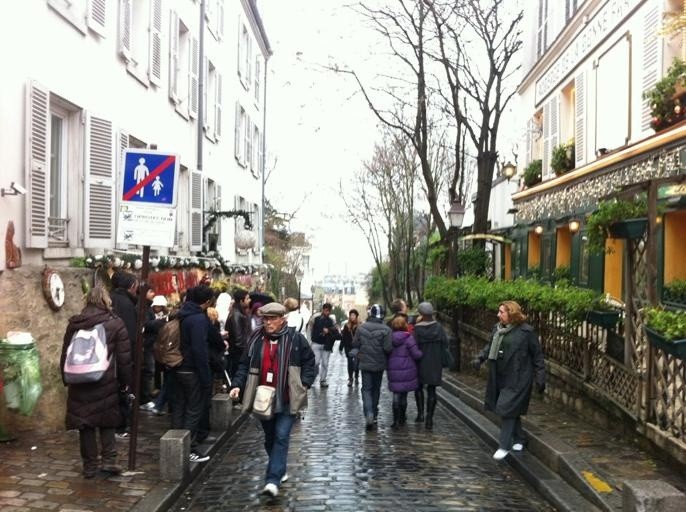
[653,57,685,107]
[641,304,685,360]
[585,193,648,259]
[584,303,618,329]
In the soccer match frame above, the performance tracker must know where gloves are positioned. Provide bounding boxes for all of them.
[471,358,482,370]
[536,382,545,393]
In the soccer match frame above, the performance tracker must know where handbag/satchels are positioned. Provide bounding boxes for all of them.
[349,347,360,370]
[441,349,456,367]
[253,385,276,421]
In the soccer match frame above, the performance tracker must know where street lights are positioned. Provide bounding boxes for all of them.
[444,189,467,375]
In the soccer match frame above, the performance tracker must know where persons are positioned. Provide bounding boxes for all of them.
[151,286,218,464]
[470,301,546,462]
[306,303,363,385]
[110,268,307,439]
[228,302,316,499]
[59,284,134,479]
[353,300,448,428]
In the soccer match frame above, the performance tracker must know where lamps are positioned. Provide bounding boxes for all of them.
[566,207,581,234]
[499,161,515,185]
[533,217,543,237]
[202,209,257,251]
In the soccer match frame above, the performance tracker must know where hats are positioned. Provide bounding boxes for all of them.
[284,298,298,308]
[350,309,358,317]
[368,304,385,319]
[192,285,212,305]
[151,295,167,307]
[418,302,433,315]
[252,302,262,310]
[258,302,287,316]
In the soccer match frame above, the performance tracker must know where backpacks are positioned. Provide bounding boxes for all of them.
[62,321,114,385]
[153,318,184,368]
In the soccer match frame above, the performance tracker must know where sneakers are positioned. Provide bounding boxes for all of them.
[512,443,523,451]
[366,416,373,430]
[321,381,329,387]
[114,433,131,438]
[493,448,509,459]
[84,464,123,477]
[140,401,155,411]
[262,483,278,497]
[189,450,210,462]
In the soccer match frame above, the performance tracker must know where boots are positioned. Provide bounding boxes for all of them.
[391,391,437,429]
[348,376,358,386]
